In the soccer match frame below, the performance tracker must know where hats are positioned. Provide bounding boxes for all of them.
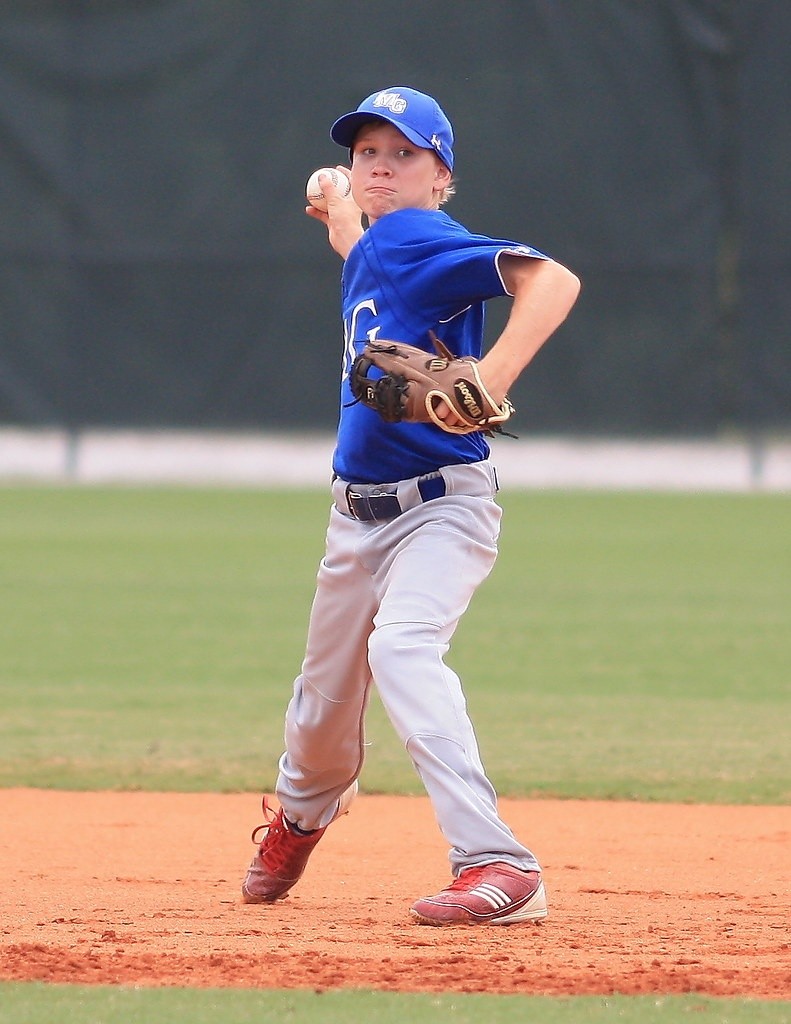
[330,86,454,170]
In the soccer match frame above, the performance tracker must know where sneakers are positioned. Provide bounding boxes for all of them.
[241,795,340,903]
[409,862,549,925]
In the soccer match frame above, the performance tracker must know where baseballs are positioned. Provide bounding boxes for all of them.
[305,168,350,214]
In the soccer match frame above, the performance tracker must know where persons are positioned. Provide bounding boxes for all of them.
[242,85,581,926]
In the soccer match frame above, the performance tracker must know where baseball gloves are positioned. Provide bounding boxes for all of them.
[345,328,510,436]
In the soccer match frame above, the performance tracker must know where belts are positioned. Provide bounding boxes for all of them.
[344,475,446,522]
